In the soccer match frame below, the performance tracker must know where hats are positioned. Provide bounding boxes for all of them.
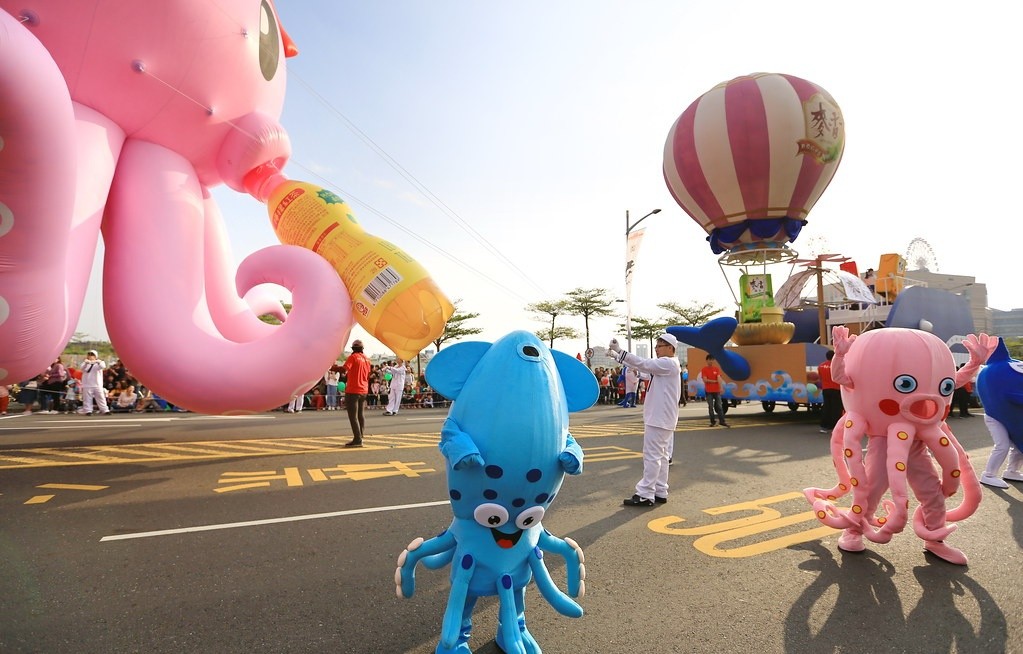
[88,350,98,358]
[67,380,76,385]
[660,333,678,352]
[351,339,365,350]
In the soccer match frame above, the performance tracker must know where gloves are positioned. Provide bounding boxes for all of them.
[604,349,619,359]
[608,338,622,354]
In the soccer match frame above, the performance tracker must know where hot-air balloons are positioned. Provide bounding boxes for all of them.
[662,70,847,324]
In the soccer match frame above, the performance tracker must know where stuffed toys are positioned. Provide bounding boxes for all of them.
[394,330,599,654]
[976,336,1023,488]
[804,325,998,566]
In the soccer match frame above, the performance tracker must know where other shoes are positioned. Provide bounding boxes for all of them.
[819,427,827,433]
[959,412,975,419]
[719,422,728,426]
[600,394,707,407]
[0,403,451,415]
[947,410,953,417]
[655,496,667,503]
[669,458,674,465]
[345,437,363,447]
[709,423,715,427]
[623,494,653,506]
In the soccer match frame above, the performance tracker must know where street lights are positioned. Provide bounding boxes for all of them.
[622,206,664,356]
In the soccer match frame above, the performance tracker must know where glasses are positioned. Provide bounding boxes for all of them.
[654,343,669,349]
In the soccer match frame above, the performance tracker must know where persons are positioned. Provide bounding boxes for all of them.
[594,363,651,408]
[702,355,729,427]
[606,333,681,506]
[283,357,451,415]
[948,362,974,419]
[818,350,844,433]
[864,269,876,292]
[0,351,189,416]
[330,340,371,446]
[678,364,695,406]
